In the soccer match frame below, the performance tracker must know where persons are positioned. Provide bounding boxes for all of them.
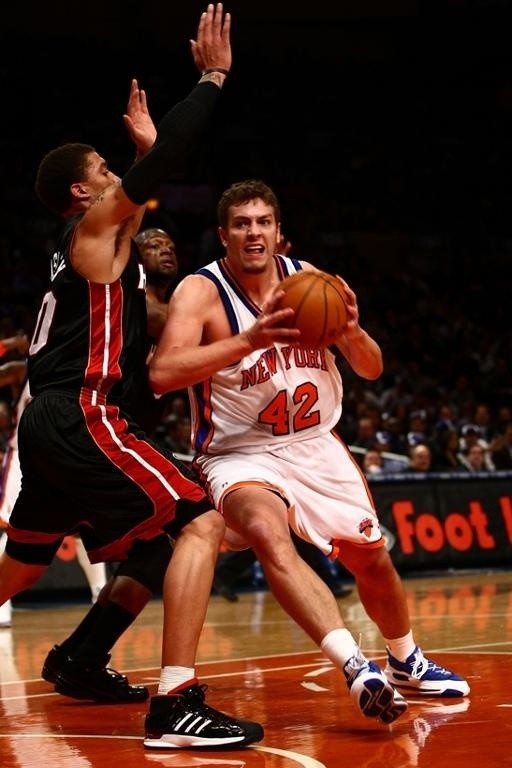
[39,221,193,702]
[0,0,271,751]
[148,174,473,724]
[1,312,511,644]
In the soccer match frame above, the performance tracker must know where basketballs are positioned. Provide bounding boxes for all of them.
[273,271,351,350]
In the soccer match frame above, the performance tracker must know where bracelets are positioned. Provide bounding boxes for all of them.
[198,65,233,80]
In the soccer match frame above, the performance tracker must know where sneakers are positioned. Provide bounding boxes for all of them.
[339,632,408,726]
[142,677,265,750]
[40,644,128,687]
[382,644,471,698]
[53,654,149,705]
